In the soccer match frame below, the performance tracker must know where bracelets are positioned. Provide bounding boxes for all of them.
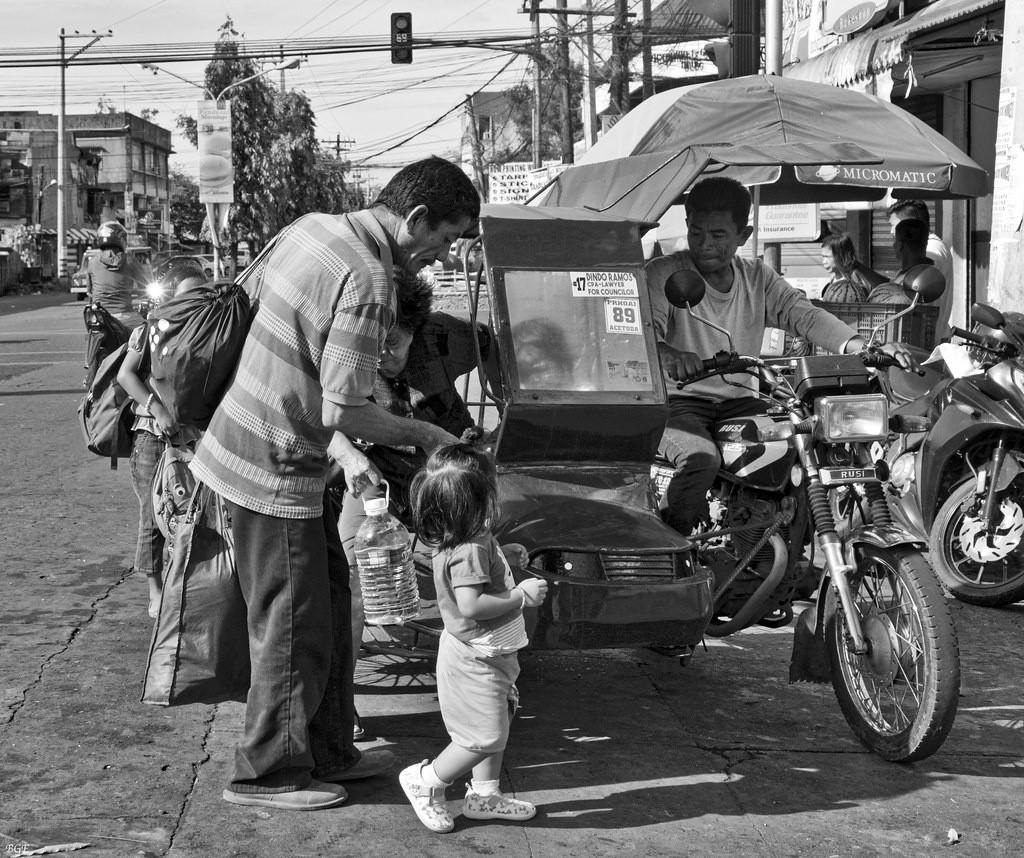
[146,393,154,413]
[512,586,525,611]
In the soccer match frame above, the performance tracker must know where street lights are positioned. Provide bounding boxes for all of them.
[37,180,57,222]
[141,59,301,283]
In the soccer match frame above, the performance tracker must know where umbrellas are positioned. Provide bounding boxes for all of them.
[572,74,990,260]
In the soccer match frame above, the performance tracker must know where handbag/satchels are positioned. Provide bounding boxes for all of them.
[141,483,247,706]
[152,432,196,543]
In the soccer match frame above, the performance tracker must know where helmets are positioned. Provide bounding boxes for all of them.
[97,221,127,252]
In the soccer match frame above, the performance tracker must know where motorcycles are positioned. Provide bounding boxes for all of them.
[873,300,1024,609]
[82,283,161,392]
[334,206,960,768]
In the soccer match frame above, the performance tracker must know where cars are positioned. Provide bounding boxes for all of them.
[153,246,254,287]
[69,245,152,301]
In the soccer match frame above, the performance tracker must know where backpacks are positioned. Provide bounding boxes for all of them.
[76,321,154,470]
[146,214,306,431]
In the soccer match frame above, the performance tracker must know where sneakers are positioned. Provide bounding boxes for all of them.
[222,777,348,811]
[398,759,454,832]
[320,749,396,781]
[462,783,536,821]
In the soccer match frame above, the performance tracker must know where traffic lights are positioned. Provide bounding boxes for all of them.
[390,12,414,64]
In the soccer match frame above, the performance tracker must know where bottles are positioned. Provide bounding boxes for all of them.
[353,478,423,624]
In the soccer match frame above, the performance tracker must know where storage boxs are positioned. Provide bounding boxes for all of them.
[810,299,941,357]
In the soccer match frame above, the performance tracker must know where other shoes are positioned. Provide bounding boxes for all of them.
[649,654,686,692]
[148,600,159,620]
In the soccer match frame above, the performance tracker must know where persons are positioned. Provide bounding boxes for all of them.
[822,199,954,347]
[644,177,921,654]
[399,443,547,834]
[86,221,151,329]
[327,264,506,739]
[511,318,604,391]
[117,267,208,619]
[188,156,481,810]
